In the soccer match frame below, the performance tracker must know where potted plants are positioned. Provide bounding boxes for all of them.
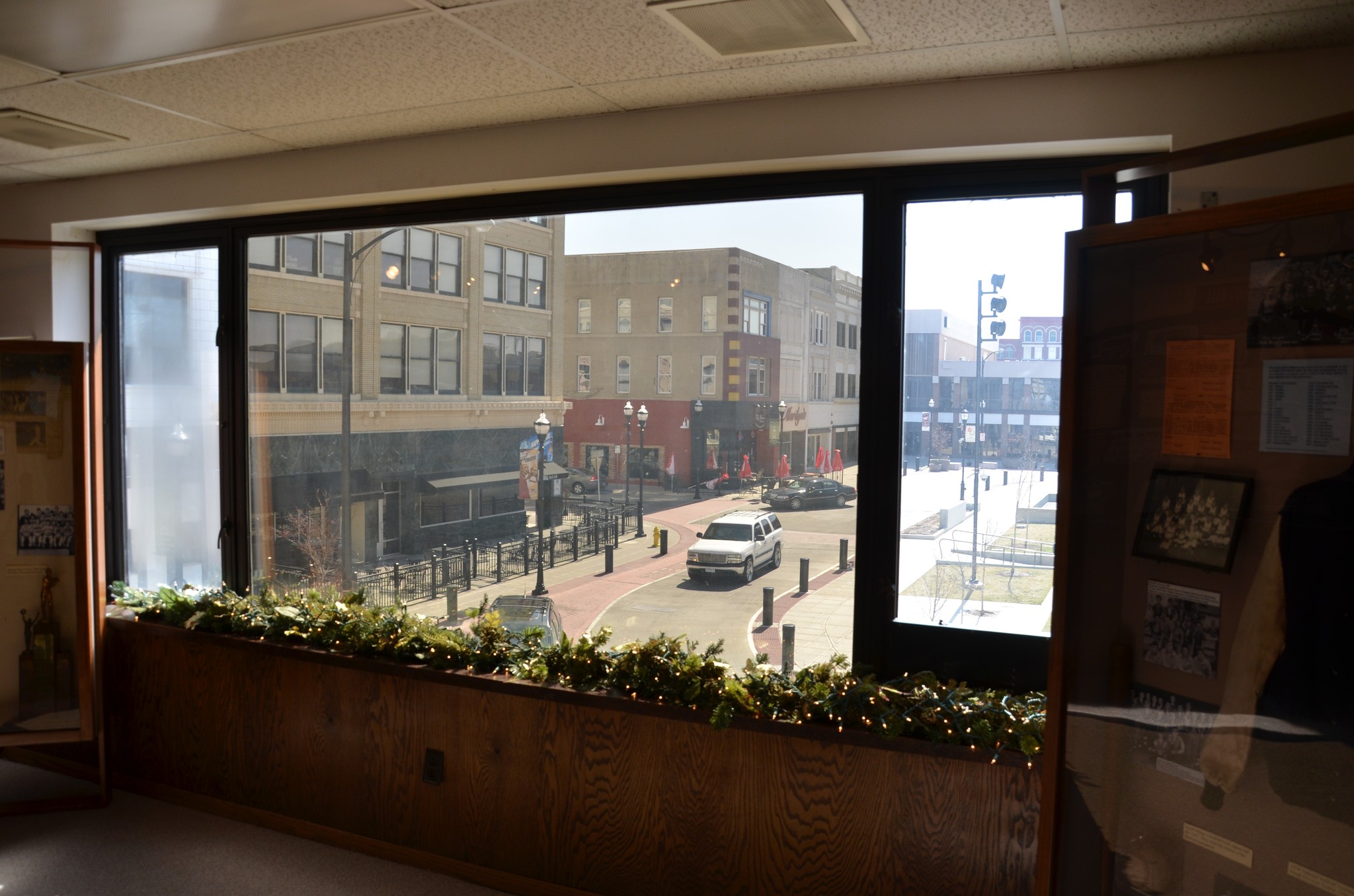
[930,423,952,470]
[998,431,1024,468]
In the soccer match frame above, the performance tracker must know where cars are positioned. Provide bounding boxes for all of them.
[761,477,857,512]
[562,467,608,495]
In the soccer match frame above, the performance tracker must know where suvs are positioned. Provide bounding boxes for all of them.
[686,509,784,585]
[473,594,565,656]
[1038,431,1055,445]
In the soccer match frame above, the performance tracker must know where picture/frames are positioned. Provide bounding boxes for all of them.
[1132,468,1255,574]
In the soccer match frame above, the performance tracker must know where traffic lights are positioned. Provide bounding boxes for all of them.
[978,274,1007,341]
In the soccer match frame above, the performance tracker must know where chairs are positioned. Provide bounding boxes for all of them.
[352,501,614,607]
[733,468,777,497]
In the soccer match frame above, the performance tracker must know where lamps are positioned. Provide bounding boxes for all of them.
[680,417,689,429]
[595,414,604,425]
[754,403,773,409]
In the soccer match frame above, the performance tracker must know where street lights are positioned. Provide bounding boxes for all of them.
[533,409,552,594]
[693,397,704,499]
[635,402,647,537]
[979,349,1005,462]
[778,398,787,487]
[928,397,934,467]
[623,399,633,517]
[960,405,969,500]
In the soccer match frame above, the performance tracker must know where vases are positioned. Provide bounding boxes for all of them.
[967,462,972,466]
[992,454,996,456]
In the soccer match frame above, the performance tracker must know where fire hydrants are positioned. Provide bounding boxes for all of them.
[651,527,661,546]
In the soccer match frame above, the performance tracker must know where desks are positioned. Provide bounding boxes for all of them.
[561,529,574,533]
[595,505,614,514]
[402,565,428,593]
[521,538,538,542]
[431,547,452,557]
[352,558,364,564]
[357,575,380,606]
[578,503,597,512]
